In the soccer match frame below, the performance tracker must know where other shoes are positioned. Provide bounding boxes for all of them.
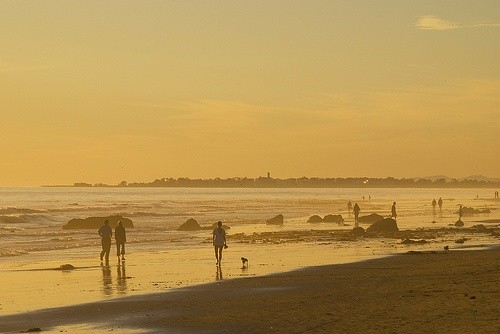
[122,257,126,260]
[219,264,221,267]
[216,262,219,265]
[118,257,120,261]
[100,253,103,261]
[106,259,109,262]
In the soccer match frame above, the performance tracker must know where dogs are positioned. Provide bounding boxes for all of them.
[240,256,249,268]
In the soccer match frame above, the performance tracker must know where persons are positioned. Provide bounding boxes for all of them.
[98,219,112,261]
[346,200,352,213]
[354,202,360,224]
[391,201,397,219]
[458,205,463,221]
[115,220,126,260]
[438,197,443,210]
[213,220,227,266]
[432,198,437,209]
